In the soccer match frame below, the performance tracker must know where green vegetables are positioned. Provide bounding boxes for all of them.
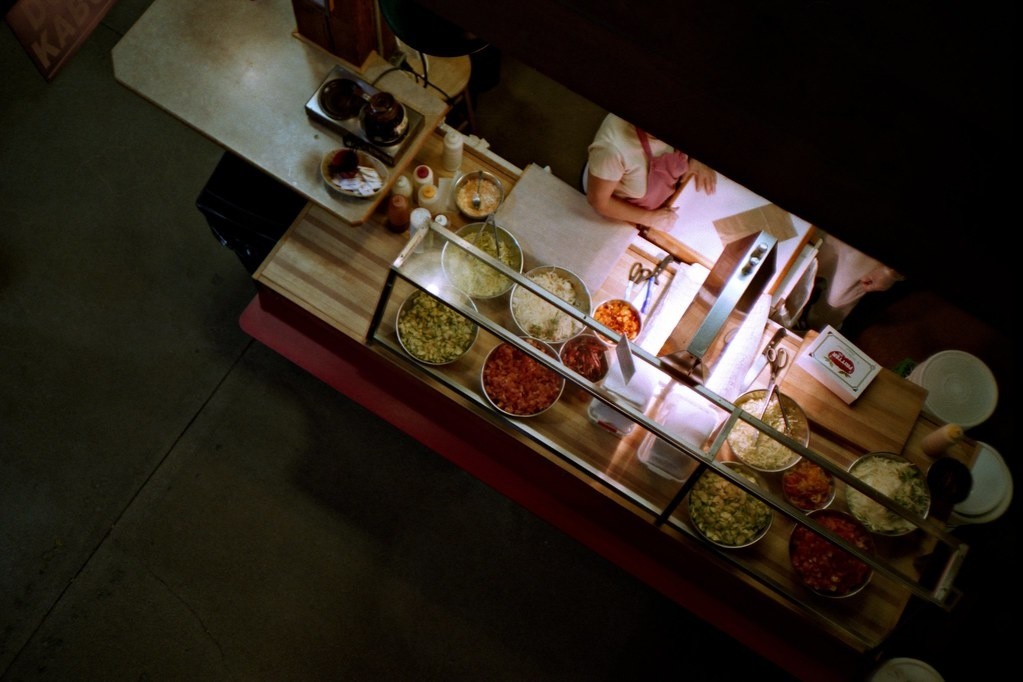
[882,470,932,523]
[690,481,771,532]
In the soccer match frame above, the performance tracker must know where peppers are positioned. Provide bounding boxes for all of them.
[561,340,608,382]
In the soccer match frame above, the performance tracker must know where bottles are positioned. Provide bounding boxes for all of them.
[742,242,768,275]
[387,165,452,234]
[442,130,464,172]
[921,423,963,458]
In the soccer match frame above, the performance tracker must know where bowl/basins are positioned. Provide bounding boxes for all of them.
[927,457,972,502]
[320,148,389,198]
[440,222,524,300]
[480,336,566,417]
[591,297,643,348]
[509,265,593,344]
[395,284,480,365]
[558,333,612,386]
[452,170,504,220]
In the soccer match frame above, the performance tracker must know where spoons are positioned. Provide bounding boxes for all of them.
[472,170,483,210]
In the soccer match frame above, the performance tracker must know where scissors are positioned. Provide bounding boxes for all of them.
[623,261,652,302]
[767,347,788,389]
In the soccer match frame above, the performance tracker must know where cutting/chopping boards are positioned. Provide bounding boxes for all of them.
[778,329,930,456]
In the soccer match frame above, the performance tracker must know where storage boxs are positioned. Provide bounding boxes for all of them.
[637,392,719,483]
[588,360,650,438]
[797,324,881,404]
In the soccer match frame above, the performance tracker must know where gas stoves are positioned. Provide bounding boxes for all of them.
[301,64,425,167]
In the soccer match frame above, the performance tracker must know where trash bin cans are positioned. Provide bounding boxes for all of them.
[195,150,308,291]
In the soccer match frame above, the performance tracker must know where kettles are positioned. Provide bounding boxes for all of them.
[359,91,409,145]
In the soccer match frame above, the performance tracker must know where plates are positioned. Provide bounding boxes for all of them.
[686,388,933,601]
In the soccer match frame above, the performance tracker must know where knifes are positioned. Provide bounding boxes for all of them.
[632,255,674,311]
[743,329,784,391]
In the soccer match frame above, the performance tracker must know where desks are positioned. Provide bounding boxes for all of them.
[644,168,817,297]
[111,1,448,225]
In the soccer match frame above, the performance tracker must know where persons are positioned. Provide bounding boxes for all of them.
[801,226,899,333]
[581,113,718,232]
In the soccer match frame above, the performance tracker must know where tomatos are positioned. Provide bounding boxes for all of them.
[483,340,564,414]
[791,515,874,597]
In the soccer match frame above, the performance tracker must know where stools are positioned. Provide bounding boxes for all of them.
[397,38,477,136]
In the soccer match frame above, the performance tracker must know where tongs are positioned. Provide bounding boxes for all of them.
[750,383,791,448]
[464,210,501,262]
